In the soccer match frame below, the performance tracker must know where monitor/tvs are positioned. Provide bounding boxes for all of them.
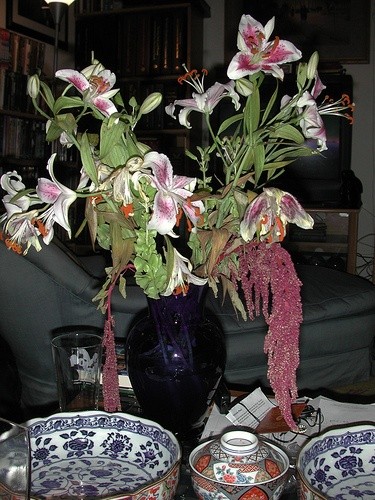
[213,73,352,209]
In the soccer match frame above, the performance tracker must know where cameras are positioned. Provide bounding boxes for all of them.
[214,381,231,414]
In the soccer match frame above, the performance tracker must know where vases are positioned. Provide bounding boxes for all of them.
[125,284,228,430]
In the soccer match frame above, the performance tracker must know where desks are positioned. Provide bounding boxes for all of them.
[59,386,375,500]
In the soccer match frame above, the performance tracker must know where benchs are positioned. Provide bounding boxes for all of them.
[0,235,375,417]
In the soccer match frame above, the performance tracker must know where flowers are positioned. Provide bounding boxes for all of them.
[0,15,354,434]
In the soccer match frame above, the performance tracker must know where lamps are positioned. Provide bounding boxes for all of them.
[44,0,76,101]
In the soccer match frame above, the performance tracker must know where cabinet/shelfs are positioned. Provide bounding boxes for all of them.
[280,207,360,273]
[0,27,82,244]
[74,0,211,245]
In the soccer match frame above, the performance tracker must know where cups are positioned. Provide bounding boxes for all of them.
[189,430,295,500]
[51,333,103,412]
[0,417,31,500]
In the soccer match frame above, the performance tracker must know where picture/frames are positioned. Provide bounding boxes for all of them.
[223,0,372,65]
[6,0,69,50]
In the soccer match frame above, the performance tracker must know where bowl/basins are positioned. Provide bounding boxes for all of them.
[0,411,182,500]
[295,421,375,500]
